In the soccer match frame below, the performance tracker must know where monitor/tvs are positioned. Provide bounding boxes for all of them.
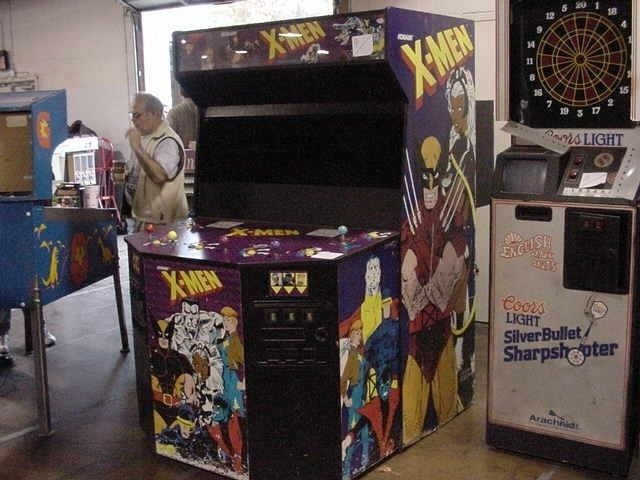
[501,156,547,196]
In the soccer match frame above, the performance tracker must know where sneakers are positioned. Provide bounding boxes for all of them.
[0,333,11,354]
[42,322,58,348]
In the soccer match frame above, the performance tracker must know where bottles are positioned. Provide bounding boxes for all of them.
[112,159,127,186]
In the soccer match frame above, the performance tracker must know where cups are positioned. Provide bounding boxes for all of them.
[85,185,100,208]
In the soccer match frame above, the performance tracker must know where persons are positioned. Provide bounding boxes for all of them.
[400,136,468,448]
[0,305,56,361]
[166,84,201,148]
[124,93,190,232]
[335,256,399,460]
[420,67,478,232]
[151,296,247,478]
